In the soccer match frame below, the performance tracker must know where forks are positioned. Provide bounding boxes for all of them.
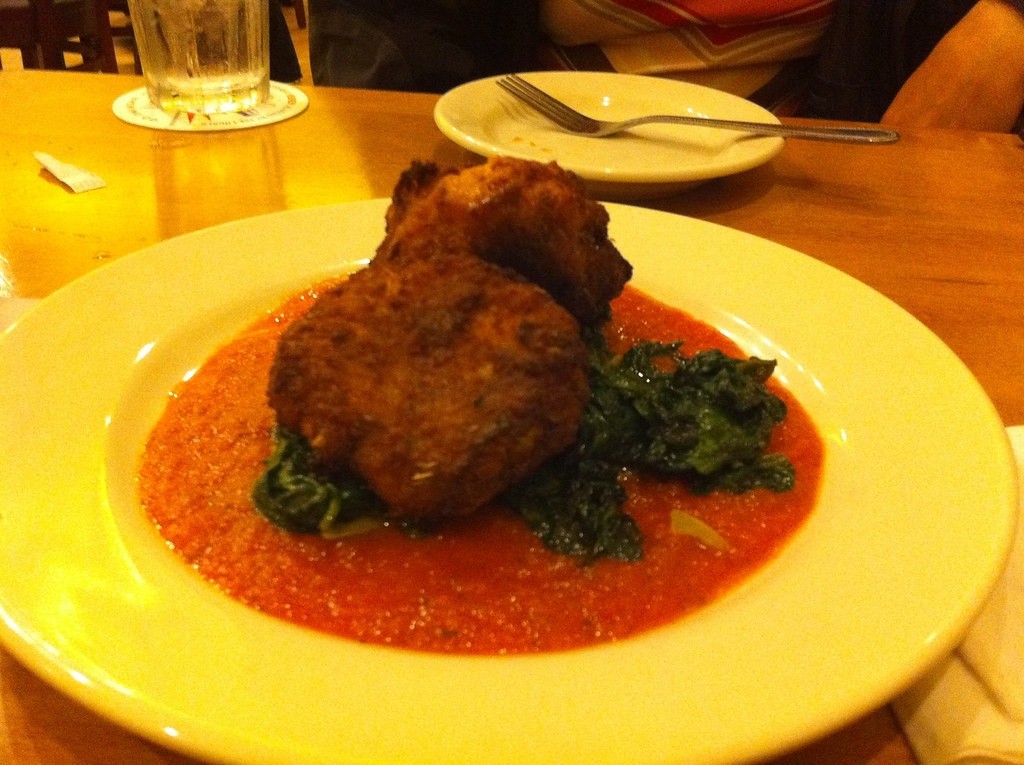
[494,73,901,146]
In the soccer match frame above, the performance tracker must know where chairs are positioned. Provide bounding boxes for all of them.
[0,0,119,74]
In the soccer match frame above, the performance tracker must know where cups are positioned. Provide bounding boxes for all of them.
[127,0,270,114]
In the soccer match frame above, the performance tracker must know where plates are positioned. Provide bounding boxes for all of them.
[432,72,785,199]
[1,199,1021,764]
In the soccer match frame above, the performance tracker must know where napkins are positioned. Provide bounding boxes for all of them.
[884,423,1024,765]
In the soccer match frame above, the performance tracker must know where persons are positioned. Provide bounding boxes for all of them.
[304,0,1024,134]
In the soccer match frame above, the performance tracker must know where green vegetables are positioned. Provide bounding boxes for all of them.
[252,327,800,563]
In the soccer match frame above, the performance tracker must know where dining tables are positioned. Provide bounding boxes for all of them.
[0,67,1024,765]
